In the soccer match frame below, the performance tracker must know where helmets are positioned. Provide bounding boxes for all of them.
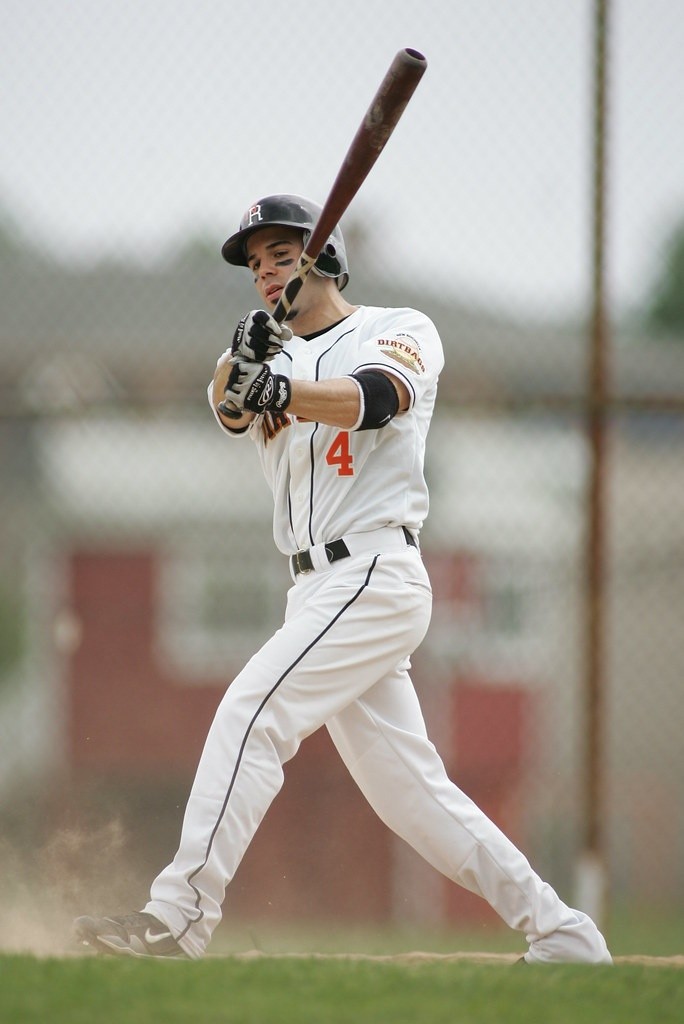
[222,194,349,293]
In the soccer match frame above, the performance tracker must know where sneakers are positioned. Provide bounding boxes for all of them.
[75,911,192,962]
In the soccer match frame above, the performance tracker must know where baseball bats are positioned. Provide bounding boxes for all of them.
[218,47,428,420]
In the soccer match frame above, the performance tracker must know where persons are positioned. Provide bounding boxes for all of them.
[70,194,615,967]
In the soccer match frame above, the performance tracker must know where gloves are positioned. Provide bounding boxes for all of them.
[231,309,294,362]
[222,355,291,416]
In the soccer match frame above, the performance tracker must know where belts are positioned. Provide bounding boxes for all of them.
[291,525,418,575]
